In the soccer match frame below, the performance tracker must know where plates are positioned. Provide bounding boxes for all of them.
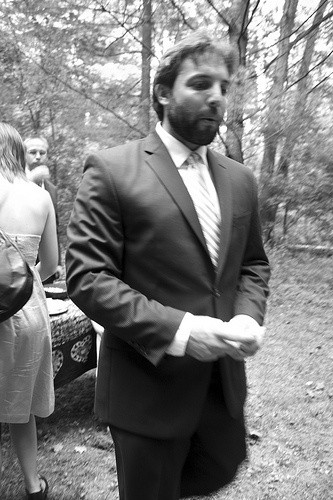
[44,298,68,315]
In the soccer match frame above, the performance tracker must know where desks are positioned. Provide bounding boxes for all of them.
[44,284,97,390]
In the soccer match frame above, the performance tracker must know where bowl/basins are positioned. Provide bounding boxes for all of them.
[42,287,68,300]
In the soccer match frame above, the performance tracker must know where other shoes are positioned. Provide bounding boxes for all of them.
[25,473,48,500]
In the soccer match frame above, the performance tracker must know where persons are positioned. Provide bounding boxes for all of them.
[65,34,274,500]
[0,121,56,500]
[22,136,58,230]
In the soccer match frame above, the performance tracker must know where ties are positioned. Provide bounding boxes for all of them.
[189,152,223,270]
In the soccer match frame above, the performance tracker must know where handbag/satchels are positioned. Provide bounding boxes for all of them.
[0,229,34,322]
